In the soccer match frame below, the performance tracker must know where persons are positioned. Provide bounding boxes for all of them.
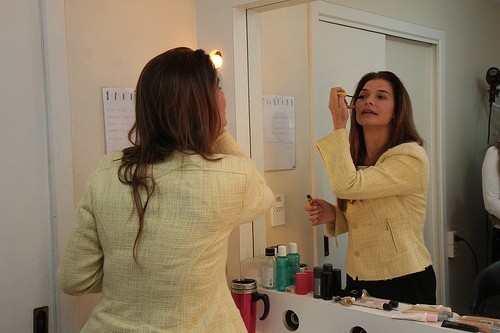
[56,47,274,333]
[482,142,500,263]
[305,71,437,306]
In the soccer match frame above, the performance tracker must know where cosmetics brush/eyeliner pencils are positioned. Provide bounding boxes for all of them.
[306,195,314,205]
[337,92,363,99]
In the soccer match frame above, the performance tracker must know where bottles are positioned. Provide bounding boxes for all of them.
[322,272,333,300]
[276,246,291,291]
[323,263,333,272]
[313,265,324,299]
[287,242,301,286]
[332,269,342,296]
[262,247,276,289]
[305,268,315,292]
[295,271,308,295]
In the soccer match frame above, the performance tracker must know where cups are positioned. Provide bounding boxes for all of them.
[230,278,270,333]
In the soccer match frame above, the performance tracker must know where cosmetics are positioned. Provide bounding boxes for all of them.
[271,242,500,326]
[243,245,500,333]
